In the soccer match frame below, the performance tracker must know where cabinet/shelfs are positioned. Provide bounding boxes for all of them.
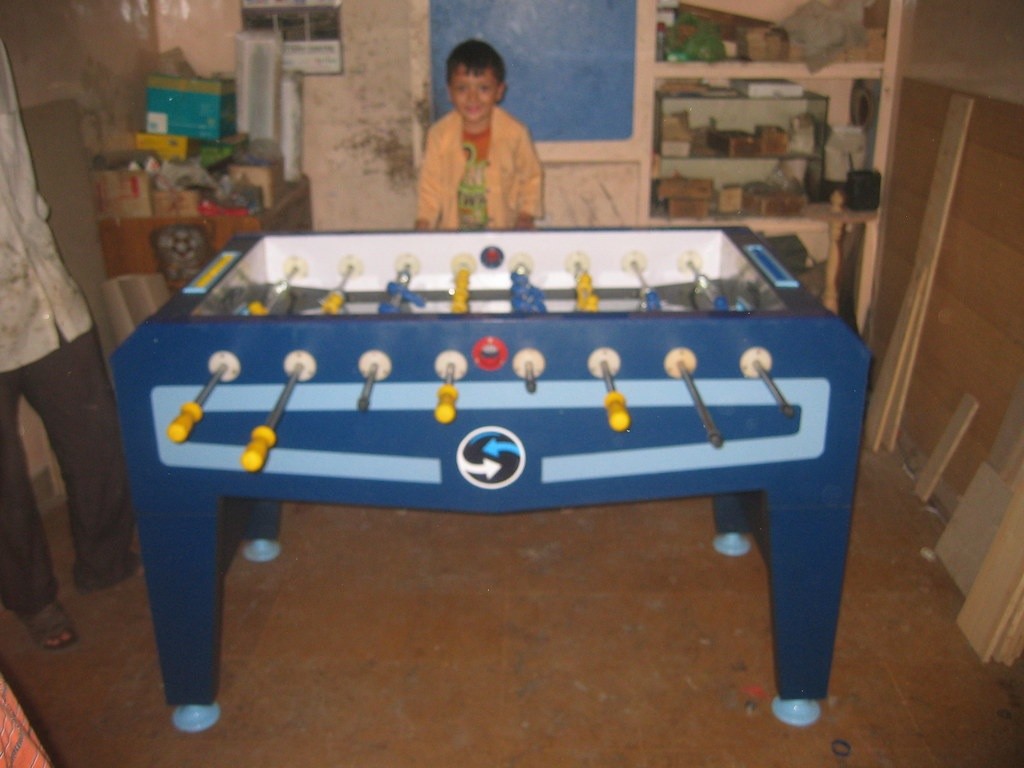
[95,174,312,293]
[637,0,904,337]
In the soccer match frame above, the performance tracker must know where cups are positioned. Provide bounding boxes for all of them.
[848,169,880,210]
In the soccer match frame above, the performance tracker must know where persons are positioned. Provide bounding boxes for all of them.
[0,38,140,656]
[414,40,546,227]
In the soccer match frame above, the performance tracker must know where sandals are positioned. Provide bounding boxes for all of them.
[121,547,147,579]
[24,599,79,652]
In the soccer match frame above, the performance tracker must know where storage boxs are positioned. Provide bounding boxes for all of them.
[660,111,821,157]
[94,48,284,218]
[655,176,806,217]
[734,31,888,61]
